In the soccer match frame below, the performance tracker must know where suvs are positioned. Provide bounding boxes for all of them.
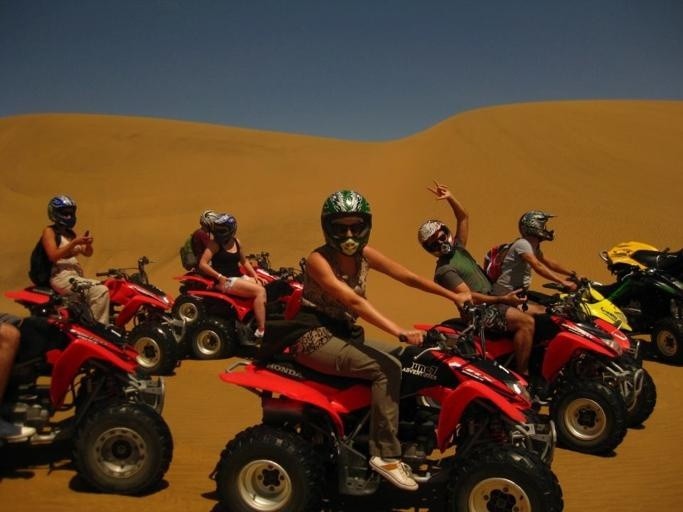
[4,254,187,378]
[170,249,312,360]
[213,301,566,511]
[536,275,644,357]
[410,290,656,457]
[0,278,178,499]
[563,240,682,365]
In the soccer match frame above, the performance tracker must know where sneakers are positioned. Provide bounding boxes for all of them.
[1,422,38,440]
[369,456,419,492]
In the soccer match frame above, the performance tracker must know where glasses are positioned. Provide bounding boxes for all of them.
[335,221,364,234]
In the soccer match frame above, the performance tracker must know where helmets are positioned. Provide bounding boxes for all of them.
[200,210,237,244]
[47,196,77,227]
[321,191,372,257]
[519,210,558,241]
[418,220,455,258]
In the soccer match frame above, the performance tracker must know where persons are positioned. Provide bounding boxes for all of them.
[483,207,578,297]
[0,309,38,441]
[40,194,110,328]
[418,178,535,376]
[192,208,219,276]
[197,212,267,339]
[291,188,473,493]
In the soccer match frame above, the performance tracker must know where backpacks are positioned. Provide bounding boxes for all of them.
[484,243,510,284]
[180,235,197,271]
[28,226,62,287]
